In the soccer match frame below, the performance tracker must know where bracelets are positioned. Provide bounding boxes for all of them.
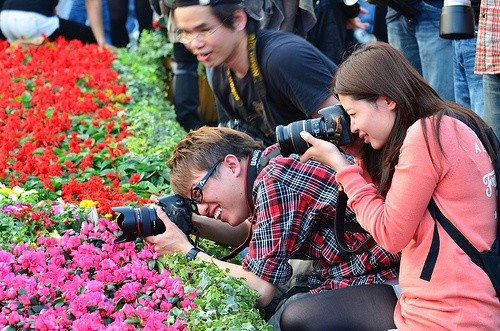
[185,247,204,262]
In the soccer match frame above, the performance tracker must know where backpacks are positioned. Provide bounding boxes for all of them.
[419,111,500,302]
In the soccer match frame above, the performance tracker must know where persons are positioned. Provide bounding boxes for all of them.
[239,0,500,140]
[171,0,339,145]
[142,123,402,311]
[279,39,500,331]
[0,0,218,133]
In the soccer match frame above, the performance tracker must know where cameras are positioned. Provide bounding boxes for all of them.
[109,194,192,244]
[276,105,359,157]
[440,0,475,39]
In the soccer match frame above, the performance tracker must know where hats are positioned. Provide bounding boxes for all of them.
[173,0,244,6]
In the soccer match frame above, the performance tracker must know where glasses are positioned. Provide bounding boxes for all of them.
[175,14,233,44]
[189,159,241,217]
